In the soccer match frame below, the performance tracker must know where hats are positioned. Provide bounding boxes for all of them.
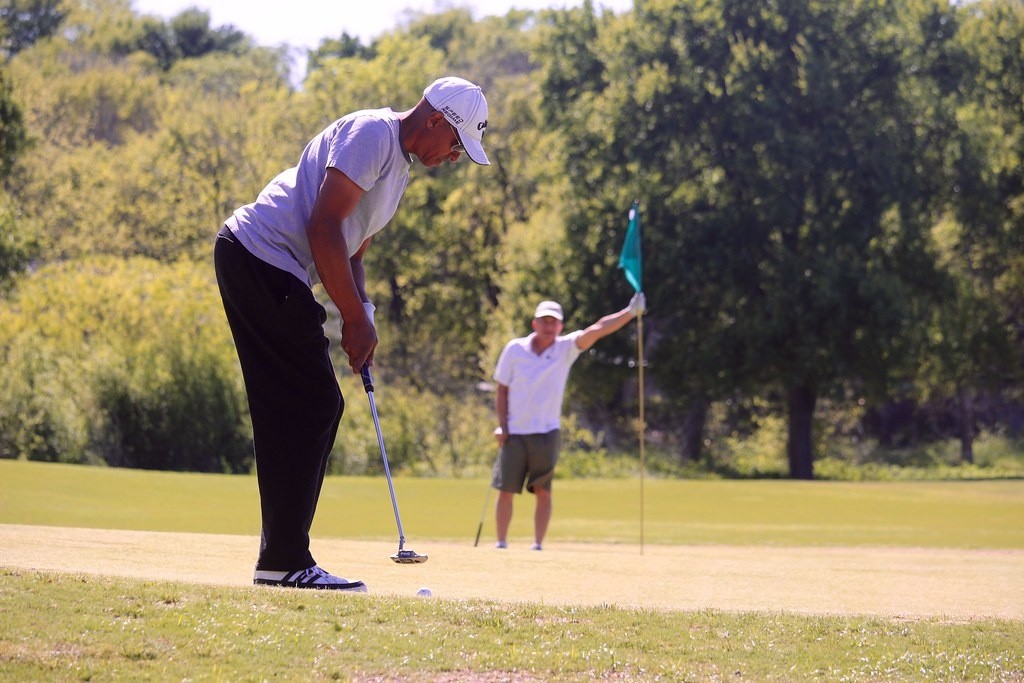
[535,300,565,320]
[423,75,490,166]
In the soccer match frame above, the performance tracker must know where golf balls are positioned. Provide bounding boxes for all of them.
[416,588,431,599]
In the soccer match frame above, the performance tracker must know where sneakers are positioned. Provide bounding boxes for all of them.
[254,562,367,592]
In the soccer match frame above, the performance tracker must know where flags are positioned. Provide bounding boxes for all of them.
[617,203,642,293]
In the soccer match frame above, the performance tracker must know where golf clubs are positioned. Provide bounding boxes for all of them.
[361,360,429,566]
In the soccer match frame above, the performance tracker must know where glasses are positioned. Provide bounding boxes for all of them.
[445,120,465,153]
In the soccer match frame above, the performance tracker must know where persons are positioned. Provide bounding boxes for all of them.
[214,77,491,590]
[490,292,646,550]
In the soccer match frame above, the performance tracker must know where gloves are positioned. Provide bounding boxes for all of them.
[626,292,645,317]
[338,302,376,332]
